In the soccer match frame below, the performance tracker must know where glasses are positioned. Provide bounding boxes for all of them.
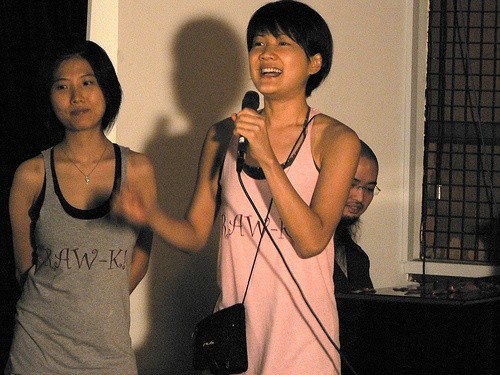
[350,185,381,195]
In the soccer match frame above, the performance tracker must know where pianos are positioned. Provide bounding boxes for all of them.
[335,277,500,375]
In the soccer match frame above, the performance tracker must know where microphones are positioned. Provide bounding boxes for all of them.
[236,90,260,173]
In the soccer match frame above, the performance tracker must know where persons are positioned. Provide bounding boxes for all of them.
[111,0,361,374]
[7,39,161,375]
[331,139,380,291]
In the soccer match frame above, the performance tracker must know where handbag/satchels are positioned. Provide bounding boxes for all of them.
[191,303,248,375]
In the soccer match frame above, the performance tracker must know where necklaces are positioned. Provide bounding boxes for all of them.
[61,139,110,183]
[253,162,261,169]
